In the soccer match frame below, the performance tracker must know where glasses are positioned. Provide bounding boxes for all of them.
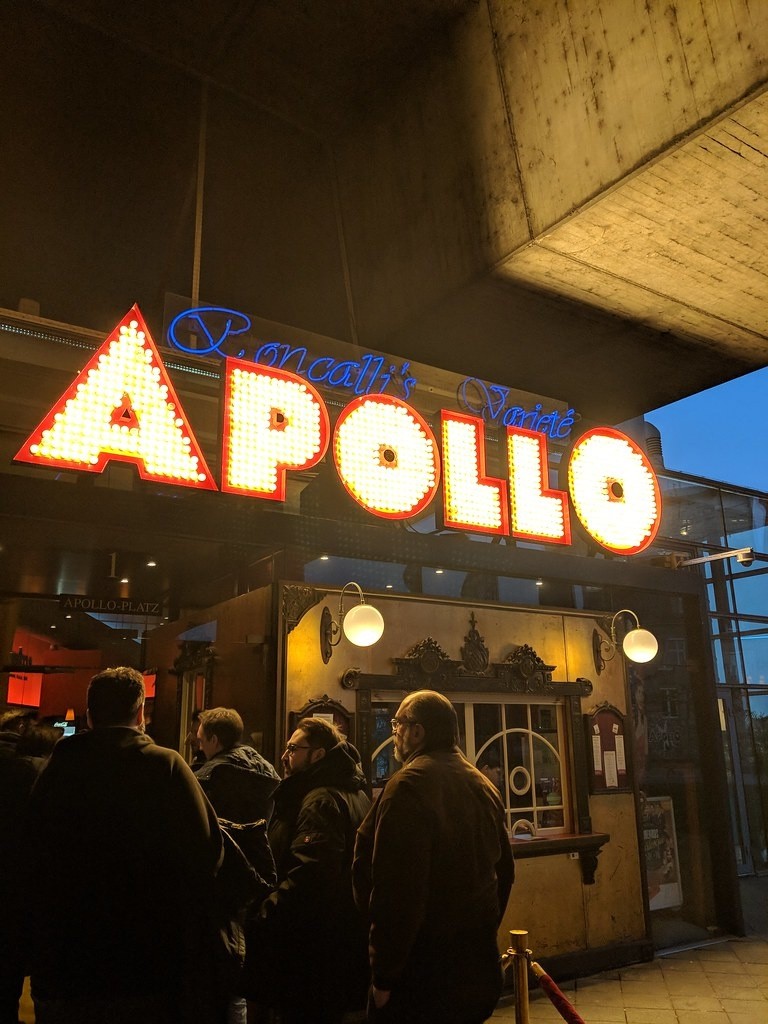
[390,718,424,731]
[285,745,313,756]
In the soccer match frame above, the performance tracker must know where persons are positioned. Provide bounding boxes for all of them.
[351,688,515,1024]
[0,667,373,1024]
[476,740,542,830]
[643,802,666,828]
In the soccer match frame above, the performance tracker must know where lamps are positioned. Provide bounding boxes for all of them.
[592,609,659,676]
[320,582,384,665]
